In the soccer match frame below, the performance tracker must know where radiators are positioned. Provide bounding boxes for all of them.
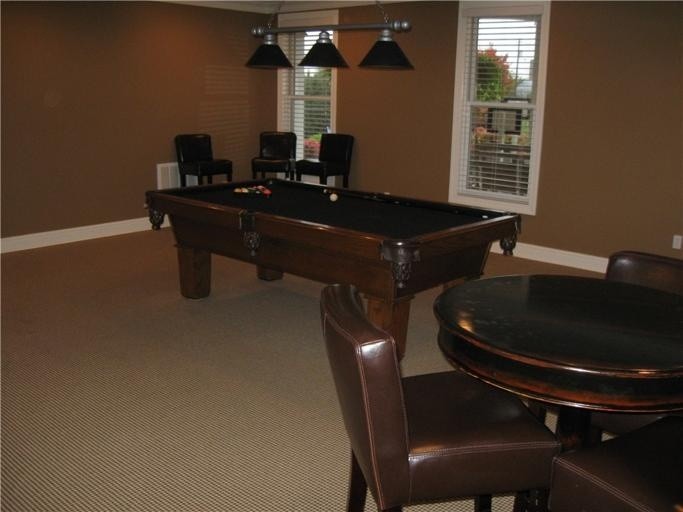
[157,162,198,190]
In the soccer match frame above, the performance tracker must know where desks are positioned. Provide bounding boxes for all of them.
[434,275,683,512]
[144,179,522,377]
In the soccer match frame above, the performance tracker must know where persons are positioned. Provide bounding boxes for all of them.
[469,106,504,191]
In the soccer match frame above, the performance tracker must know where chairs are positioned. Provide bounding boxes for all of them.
[588,249,683,445]
[250,131,297,181]
[175,133,232,187]
[295,133,355,190]
[318,283,563,512]
[547,410,683,512]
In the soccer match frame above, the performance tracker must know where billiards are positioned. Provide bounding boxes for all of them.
[234,185,272,195]
[329,194,337,202]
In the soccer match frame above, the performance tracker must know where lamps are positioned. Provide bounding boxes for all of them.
[244,1,417,71]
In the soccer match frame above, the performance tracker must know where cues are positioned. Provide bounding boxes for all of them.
[322,187,489,220]
